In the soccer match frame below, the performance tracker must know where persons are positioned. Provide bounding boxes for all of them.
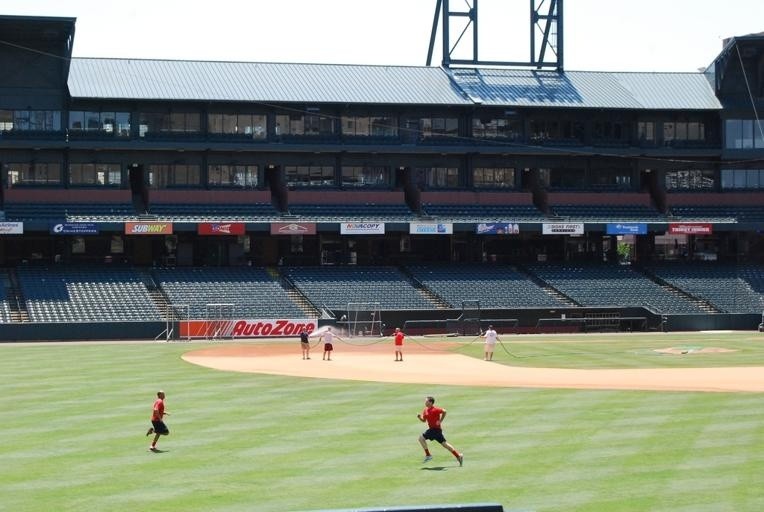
[319,327,336,360]
[479,324,500,362]
[418,397,463,469]
[393,327,404,361]
[146,391,171,451]
[301,327,311,360]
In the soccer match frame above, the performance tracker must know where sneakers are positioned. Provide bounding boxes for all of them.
[457,453,464,467]
[150,445,156,450]
[423,456,432,463]
[146,428,154,436]
